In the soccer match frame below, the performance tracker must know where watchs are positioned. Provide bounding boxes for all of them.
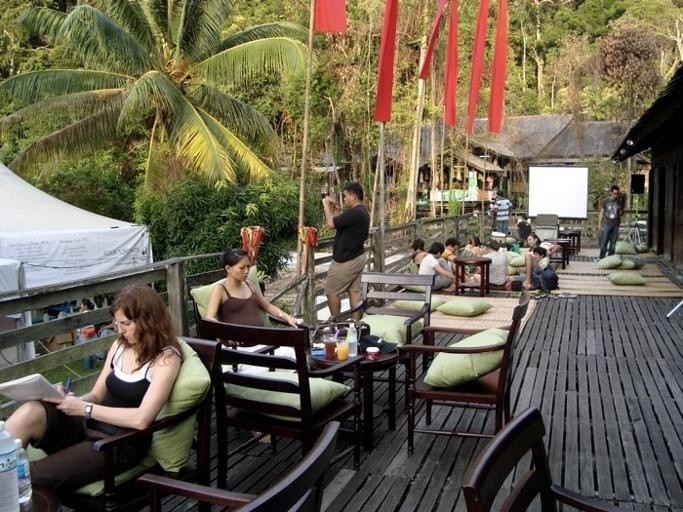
[83,400,94,420]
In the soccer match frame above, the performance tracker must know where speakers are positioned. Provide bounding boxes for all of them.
[632,175,645,194]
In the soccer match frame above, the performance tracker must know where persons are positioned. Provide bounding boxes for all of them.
[594,185,625,261]
[98,321,117,337]
[205,248,305,445]
[412,234,562,292]
[491,190,513,233]
[3,284,182,495]
[321,181,371,322]
[74,295,100,343]
[517,216,531,247]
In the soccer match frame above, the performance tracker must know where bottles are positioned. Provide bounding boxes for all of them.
[13,438,32,504]
[346,323,357,356]
[0,419,19,512]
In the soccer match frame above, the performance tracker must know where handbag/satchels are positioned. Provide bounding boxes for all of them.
[84,328,95,337]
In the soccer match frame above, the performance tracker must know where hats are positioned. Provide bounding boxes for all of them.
[483,239,499,250]
[361,335,398,353]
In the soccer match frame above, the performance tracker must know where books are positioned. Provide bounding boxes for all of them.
[0,372,66,404]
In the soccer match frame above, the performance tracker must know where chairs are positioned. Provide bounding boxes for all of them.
[197,319,366,512]
[328,274,437,414]
[25,338,221,511]
[190,282,311,354]
[398,293,529,453]
[137,420,340,512]
[459,405,620,511]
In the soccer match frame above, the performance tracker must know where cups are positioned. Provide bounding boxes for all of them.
[366,347,378,360]
[336,338,347,359]
[322,333,335,357]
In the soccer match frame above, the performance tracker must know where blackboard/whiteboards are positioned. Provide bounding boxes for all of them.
[527,164,590,220]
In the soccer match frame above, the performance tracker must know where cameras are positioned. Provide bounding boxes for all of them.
[492,196,498,200]
[328,198,333,204]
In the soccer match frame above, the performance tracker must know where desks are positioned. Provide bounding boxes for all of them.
[305,347,398,452]
[542,228,581,270]
[453,256,492,297]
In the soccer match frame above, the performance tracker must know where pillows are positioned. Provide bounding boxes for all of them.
[506,251,526,274]
[595,240,644,270]
[224,371,349,421]
[405,262,418,272]
[435,300,493,316]
[148,338,211,473]
[606,269,646,285]
[423,328,509,387]
[359,313,422,347]
[392,297,444,312]
[402,284,427,292]
[191,266,272,327]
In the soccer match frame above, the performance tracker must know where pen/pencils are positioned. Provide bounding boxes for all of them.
[65,377,72,394]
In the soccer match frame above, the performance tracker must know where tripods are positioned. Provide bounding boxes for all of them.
[622,194,643,247]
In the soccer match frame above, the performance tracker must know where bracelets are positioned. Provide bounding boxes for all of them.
[276,311,286,321]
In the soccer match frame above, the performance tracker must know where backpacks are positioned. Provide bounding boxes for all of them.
[532,265,559,291]
